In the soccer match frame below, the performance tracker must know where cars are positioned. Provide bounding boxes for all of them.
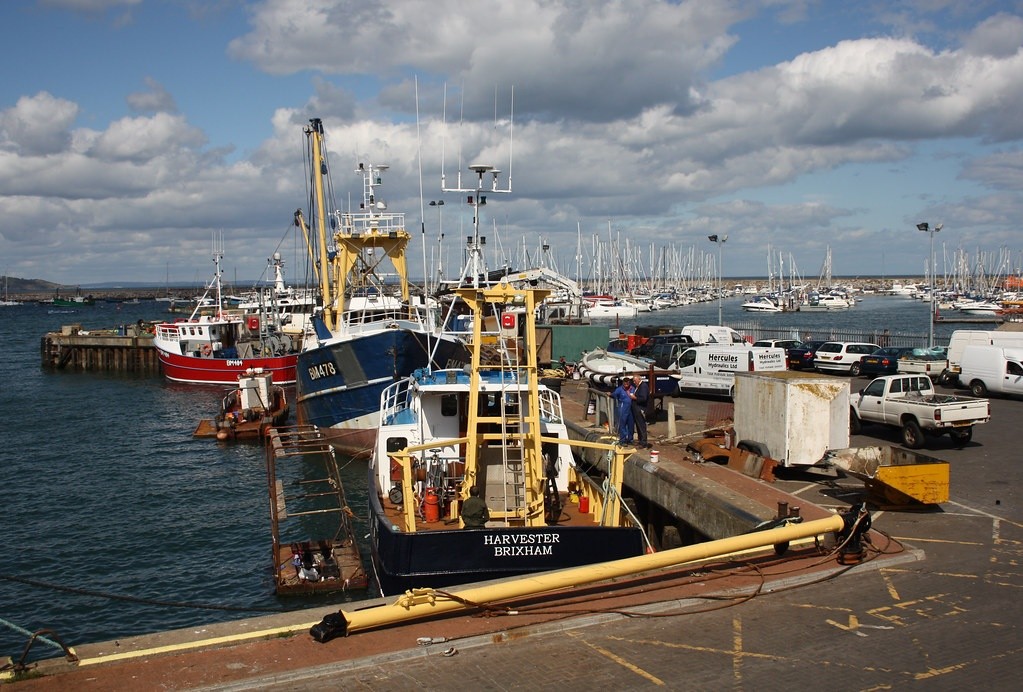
[858,346,915,380]
[750,338,810,357]
[812,342,886,377]
[788,340,829,370]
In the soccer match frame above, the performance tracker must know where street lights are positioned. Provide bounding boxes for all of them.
[917,220,947,348]
[707,234,730,326]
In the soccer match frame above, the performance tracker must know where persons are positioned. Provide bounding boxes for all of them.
[606,378,635,447]
[630,374,651,449]
[461,486,489,530]
[560,356,566,372]
[879,329,892,348]
[231,412,238,424]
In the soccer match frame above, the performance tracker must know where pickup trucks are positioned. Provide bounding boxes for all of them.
[848,374,992,450]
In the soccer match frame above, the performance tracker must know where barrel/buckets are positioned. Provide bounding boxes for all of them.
[628,335,641,351]
[578,496,589,513]
[572,371,581,380]
[651,451,659,463]
[118,324,126,335]
[642,338,649,344]
[587,401,595,415]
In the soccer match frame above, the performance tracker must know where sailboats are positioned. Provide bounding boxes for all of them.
[0,264,25,307]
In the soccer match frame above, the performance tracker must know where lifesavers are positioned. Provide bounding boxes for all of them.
[202,344,212,357]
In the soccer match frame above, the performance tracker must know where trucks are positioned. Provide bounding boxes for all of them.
[896,345,951,386]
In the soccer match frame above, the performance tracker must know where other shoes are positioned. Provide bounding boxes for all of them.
[635,444,648,451]
[628,440,634,445]
[619,441,628,447]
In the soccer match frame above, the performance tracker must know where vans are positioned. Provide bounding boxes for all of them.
[665,345,787,402]
[945,330,1023,389]
[958,345,1023,400]
[605,319,756,367]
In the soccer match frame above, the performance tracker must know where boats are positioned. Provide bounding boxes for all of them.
[576,346,682,396]
[369,75,650,584]
[194,365,293,451]
[150,212,1023,386]
[121,298,143,305]
[38,298,55,304]
[284,112,484,463]
[52,295,98,307]
[266,424,370,595]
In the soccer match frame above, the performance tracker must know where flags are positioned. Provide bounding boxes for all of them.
[629,335,649,350]
